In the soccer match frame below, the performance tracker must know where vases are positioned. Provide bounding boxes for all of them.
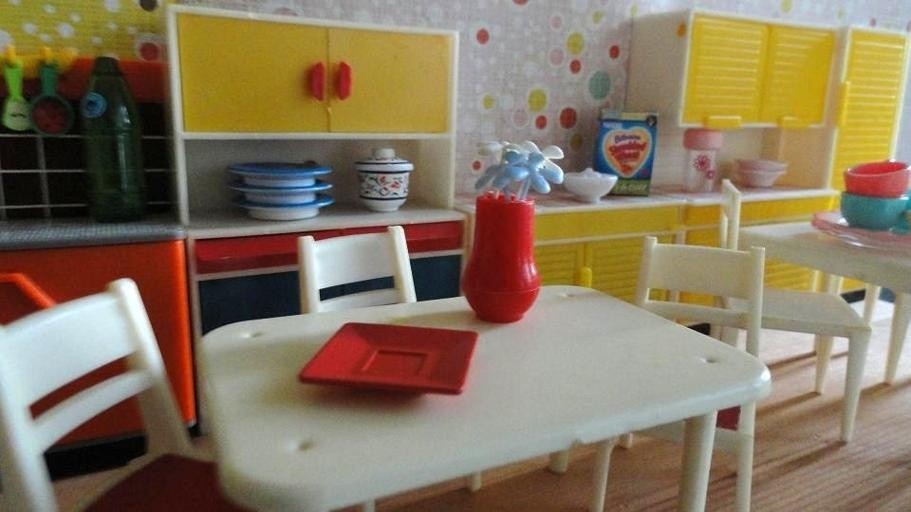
[460,193,542,324]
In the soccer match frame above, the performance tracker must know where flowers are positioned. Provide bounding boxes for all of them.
[472,138,569,204]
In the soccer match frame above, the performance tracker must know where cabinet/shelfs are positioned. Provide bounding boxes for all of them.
[821,29,909,295]
[1,134,174,221]
[534,237,667,321]
[682,15,837,131]
[174,16,453,138]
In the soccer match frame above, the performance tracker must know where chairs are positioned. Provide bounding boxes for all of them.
[716,177,872,446]
[616,234,768,511]
[0,276,252,512]
[296,222,482,512]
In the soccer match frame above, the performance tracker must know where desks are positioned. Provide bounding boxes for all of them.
[198,285,774,512]
[739,220,911,385]
